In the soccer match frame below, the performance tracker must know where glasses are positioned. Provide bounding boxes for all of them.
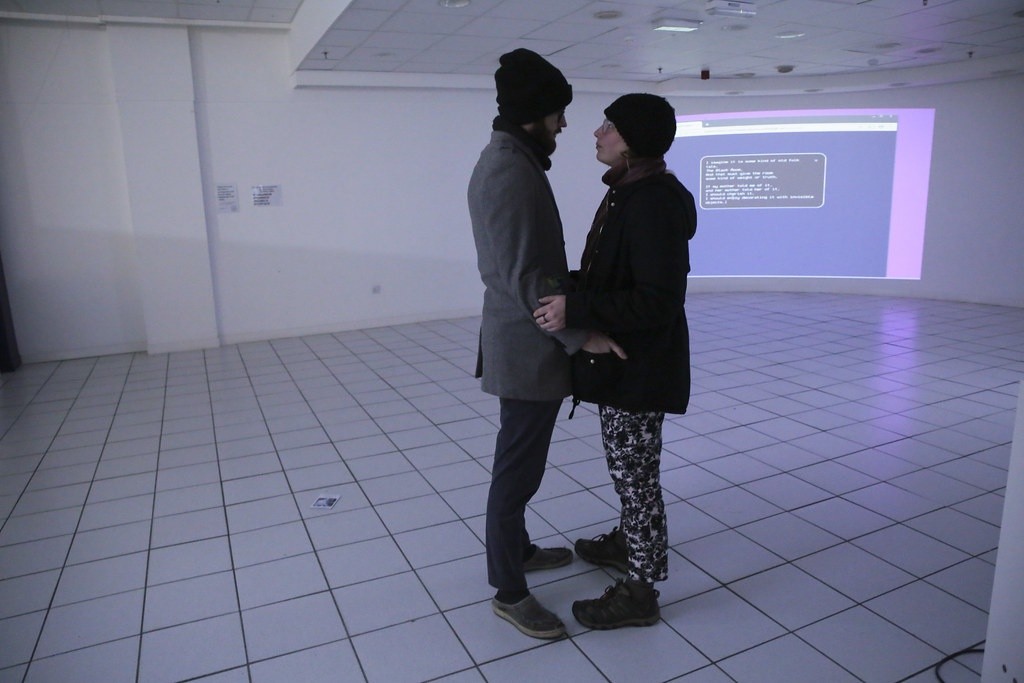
[602,119,617,134]
[551,110,567,123]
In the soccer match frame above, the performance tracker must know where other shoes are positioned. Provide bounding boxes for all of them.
[492,593,566,639]
[521,543,574,572]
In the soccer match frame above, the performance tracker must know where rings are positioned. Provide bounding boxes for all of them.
[543,315,548,322]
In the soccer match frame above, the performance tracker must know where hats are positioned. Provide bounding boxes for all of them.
[494,47,572,125]
[604,92,677,157]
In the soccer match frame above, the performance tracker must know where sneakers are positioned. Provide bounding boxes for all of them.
[571,576,661,630]
[575,524,628,573]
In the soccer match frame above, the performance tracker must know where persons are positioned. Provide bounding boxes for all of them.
[534,94,697,629]
[467,47,627,639]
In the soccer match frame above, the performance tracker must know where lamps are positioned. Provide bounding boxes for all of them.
[651,18,701,32]
[705,1,757,19]
